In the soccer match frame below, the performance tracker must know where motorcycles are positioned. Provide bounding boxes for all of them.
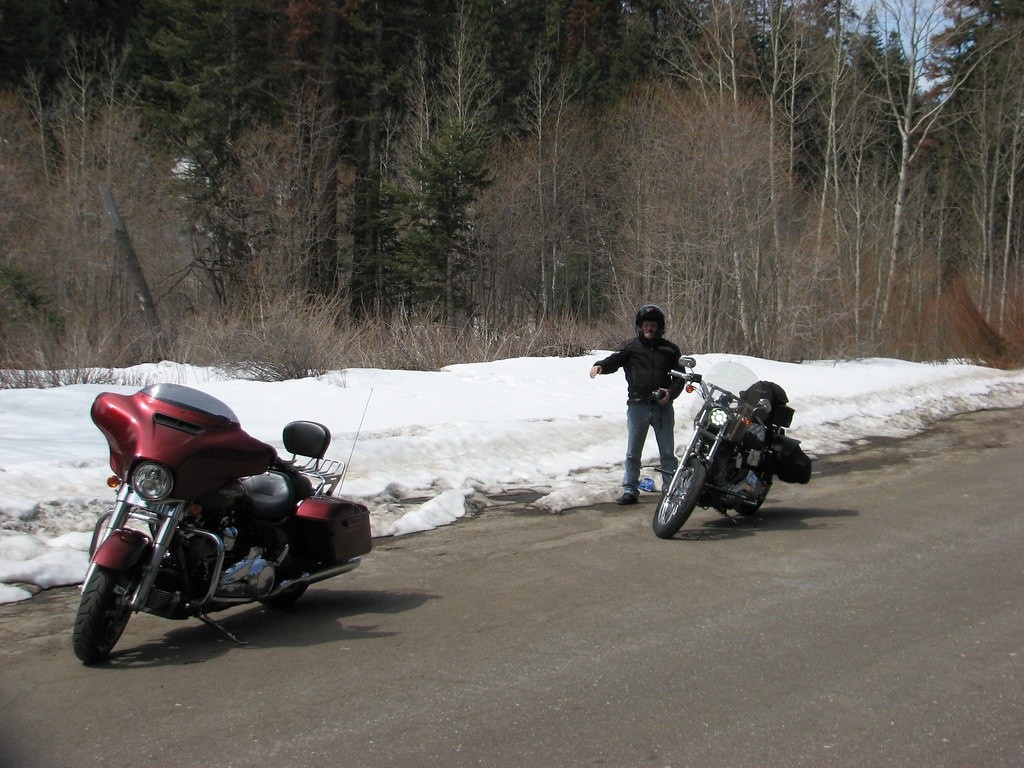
[652,355,812,539]
[72,383,375,663]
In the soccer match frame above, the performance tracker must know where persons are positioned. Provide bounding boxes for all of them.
[589,304,688,505]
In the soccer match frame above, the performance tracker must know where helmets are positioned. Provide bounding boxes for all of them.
[635,305,665,345]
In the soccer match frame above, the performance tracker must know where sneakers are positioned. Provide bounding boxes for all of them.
[617,493,638,505]
